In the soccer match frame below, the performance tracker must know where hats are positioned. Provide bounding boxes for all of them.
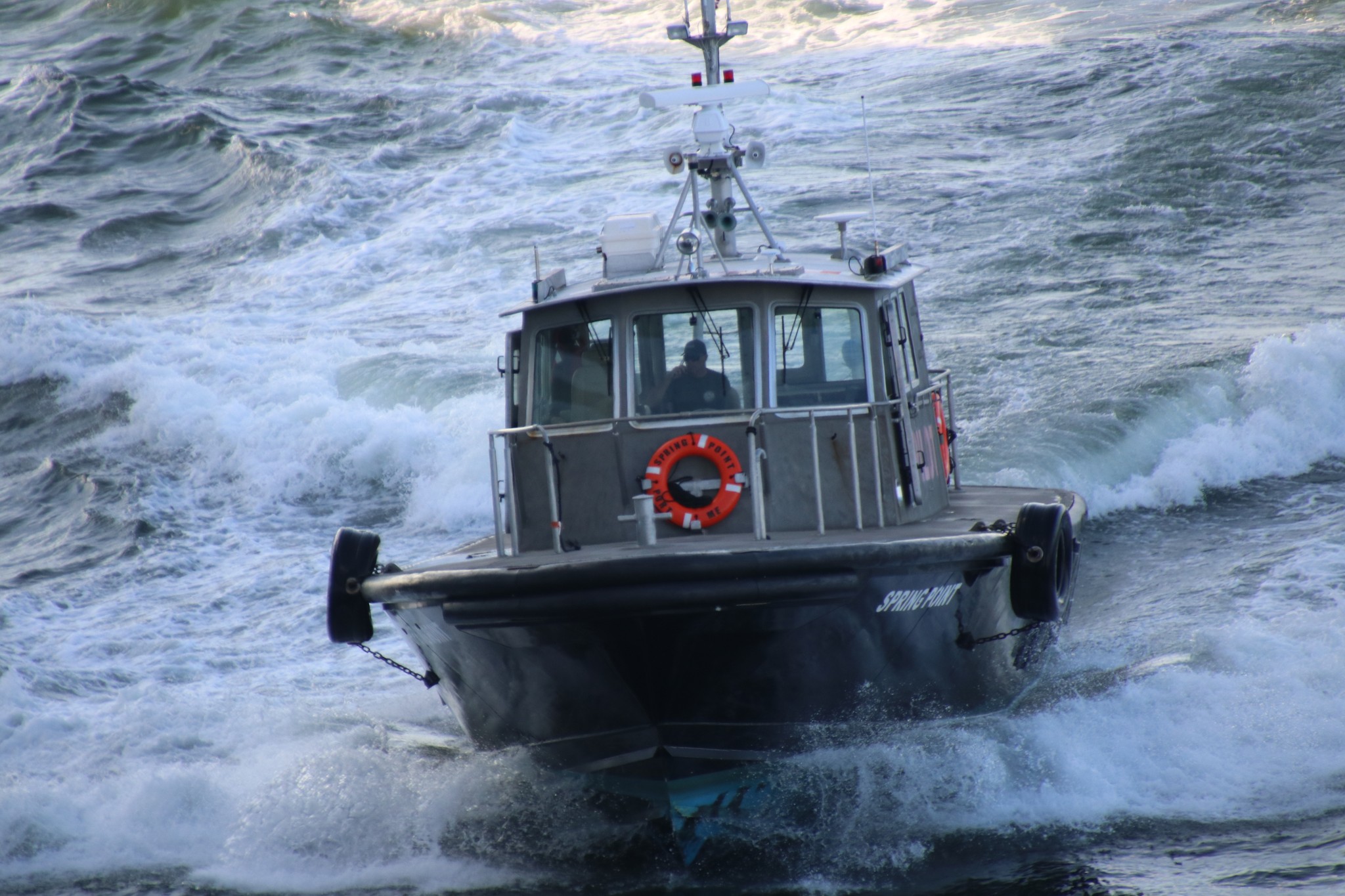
[680,339,707,356]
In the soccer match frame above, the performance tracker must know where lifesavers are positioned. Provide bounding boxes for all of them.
[932,394,951,483]
[1008,501,1078,625]
[644,431,744,530]
[325,527,381,644]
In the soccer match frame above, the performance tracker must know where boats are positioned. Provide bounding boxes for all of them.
[328,0,1088,751]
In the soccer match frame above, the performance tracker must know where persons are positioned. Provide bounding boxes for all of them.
[652,340,739,414]
[548,331,612,420]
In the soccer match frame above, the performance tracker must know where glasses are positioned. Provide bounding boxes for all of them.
[683,355,699,361]
